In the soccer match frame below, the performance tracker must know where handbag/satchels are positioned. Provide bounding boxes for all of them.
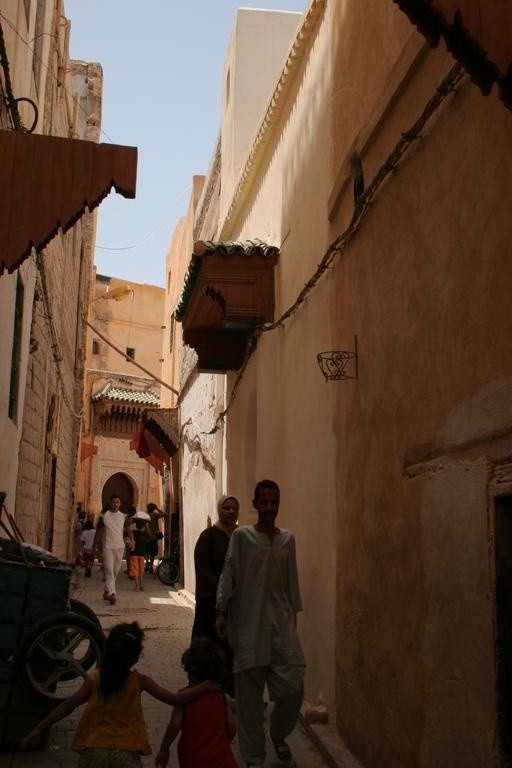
[157,531,164,540]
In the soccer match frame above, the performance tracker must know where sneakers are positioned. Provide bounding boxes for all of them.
[102,589,109,600]
[143,562,154,575]
[108,593,117,606]
[84,568,92,578]
[271,737,297,768]
[241,755,266,768]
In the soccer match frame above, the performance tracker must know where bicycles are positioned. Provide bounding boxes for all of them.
[152,545,181,587]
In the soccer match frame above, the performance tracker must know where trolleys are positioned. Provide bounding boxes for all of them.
[0,487,112,756]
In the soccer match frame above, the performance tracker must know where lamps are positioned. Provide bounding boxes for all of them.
[89,284,133,304]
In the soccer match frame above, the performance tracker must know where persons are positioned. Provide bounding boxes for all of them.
[212,478,306,767]
[188,494,240,670]
[18,621,214,768]
[74,493,166,606]
[155,637,242,768]
